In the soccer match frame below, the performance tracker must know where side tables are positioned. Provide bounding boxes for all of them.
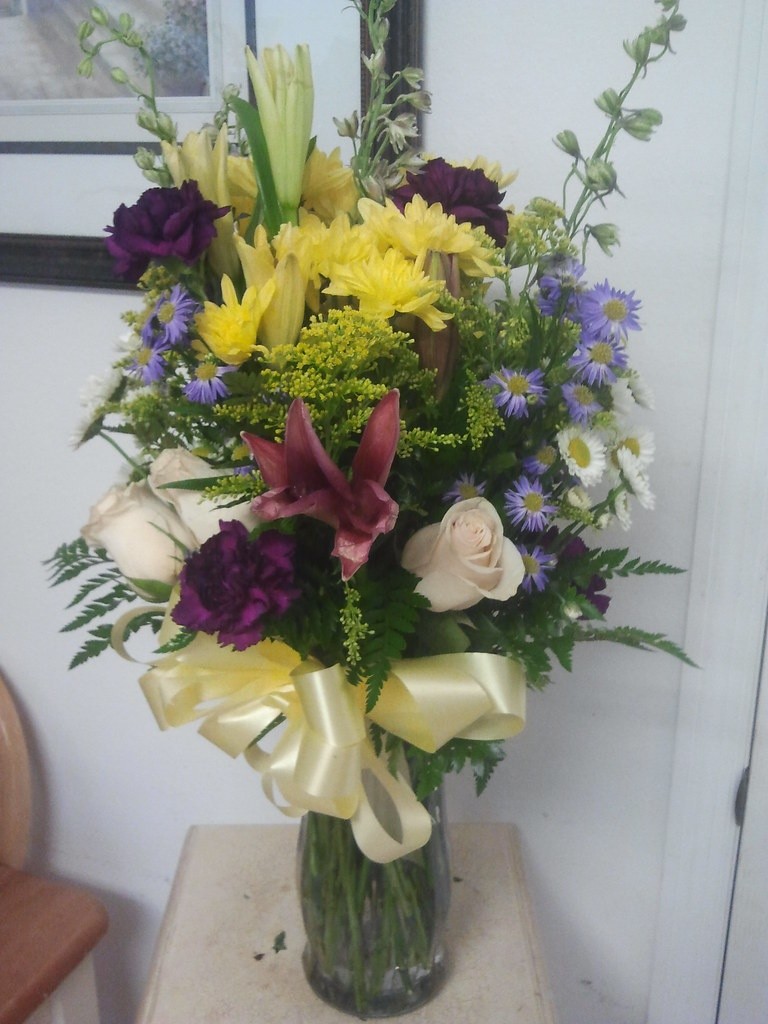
[136,823,554,1023]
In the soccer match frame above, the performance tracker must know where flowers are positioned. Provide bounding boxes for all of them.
[43,0,696,864]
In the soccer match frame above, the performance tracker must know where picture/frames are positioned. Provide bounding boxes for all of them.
[0,0,256,291]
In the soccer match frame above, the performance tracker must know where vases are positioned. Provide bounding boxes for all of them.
[296,718,456,1018]
